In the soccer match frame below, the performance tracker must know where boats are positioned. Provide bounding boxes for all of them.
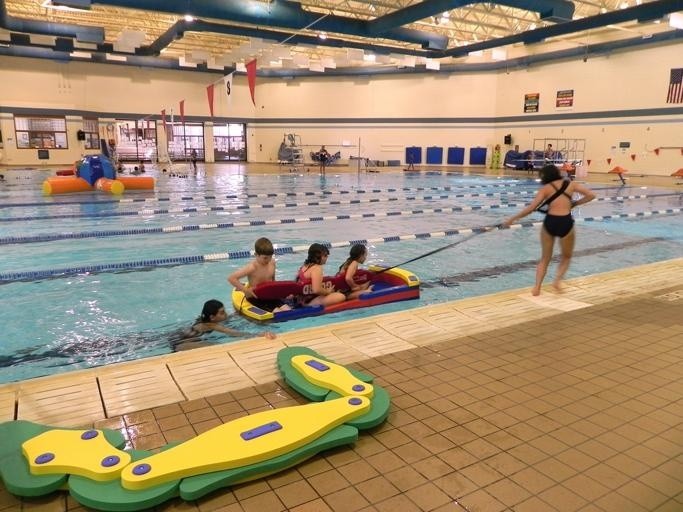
[231,262,420,325]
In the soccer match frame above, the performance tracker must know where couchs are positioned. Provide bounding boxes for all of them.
[503,150,561,168]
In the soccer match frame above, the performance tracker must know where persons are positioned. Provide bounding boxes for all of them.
[506,164,596,296]
[544,143,555,158]
[333,244,372,301]
[116,160,126,174]
[318,149,328,173]
[170,298,277,353]
[226,234,291,314]
[128,167,142,176]
[287,242,346,309]
[0,174,7,181]
[139,160,146,172]
[187,148,199,169]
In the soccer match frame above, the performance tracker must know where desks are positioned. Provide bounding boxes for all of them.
[516,157,579,175]
